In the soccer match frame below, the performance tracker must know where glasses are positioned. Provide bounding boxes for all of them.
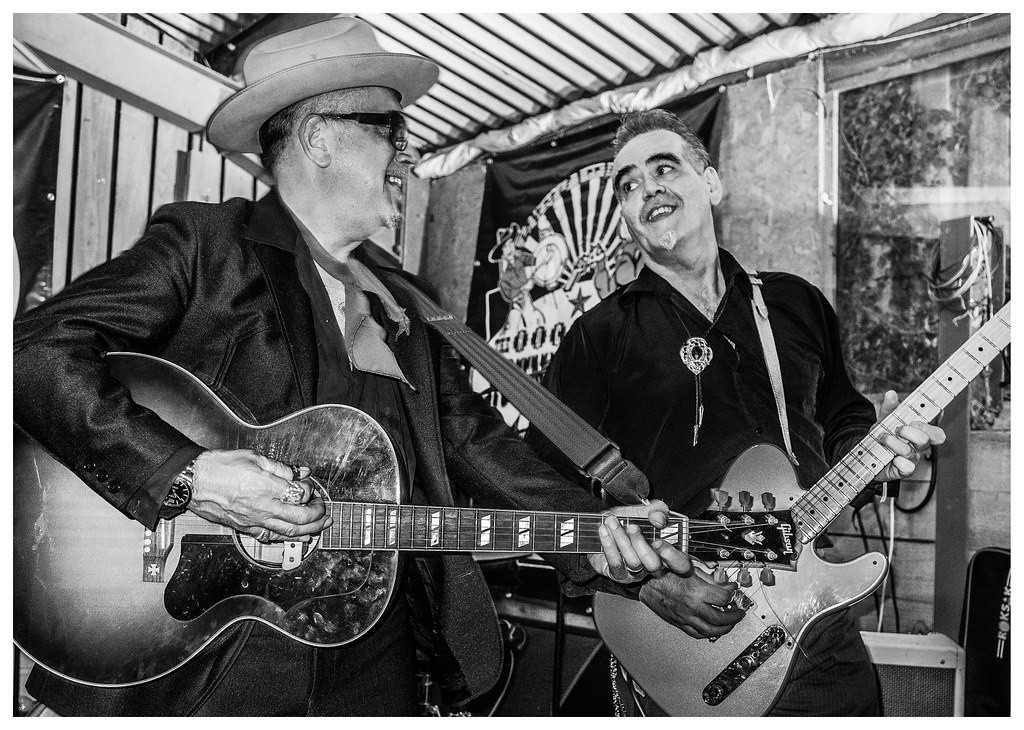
[311,110,409,152]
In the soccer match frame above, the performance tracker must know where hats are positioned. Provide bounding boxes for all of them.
[204,18,439,151]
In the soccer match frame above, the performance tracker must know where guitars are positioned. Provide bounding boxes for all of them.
[15,295,1012,716]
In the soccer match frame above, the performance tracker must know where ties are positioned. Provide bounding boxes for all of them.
[288,206,418,394]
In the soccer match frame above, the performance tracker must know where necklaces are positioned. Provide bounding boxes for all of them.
[670,301,726,447]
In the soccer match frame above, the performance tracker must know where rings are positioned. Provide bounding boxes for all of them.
[281,480,304,506]
[625,567,645,574]
[257,529,270,544]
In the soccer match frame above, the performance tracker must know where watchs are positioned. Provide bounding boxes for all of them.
[163,459,197,507]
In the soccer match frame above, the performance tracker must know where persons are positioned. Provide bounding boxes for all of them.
[15,16,693,716]
[524,110,947,717]
